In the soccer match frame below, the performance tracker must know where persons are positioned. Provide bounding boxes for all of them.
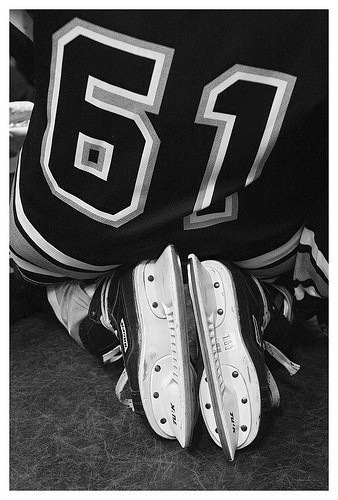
[9,10,330,462]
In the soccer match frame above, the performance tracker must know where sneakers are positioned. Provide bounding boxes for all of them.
[79,245,200,445]
[180,250,299,462]
